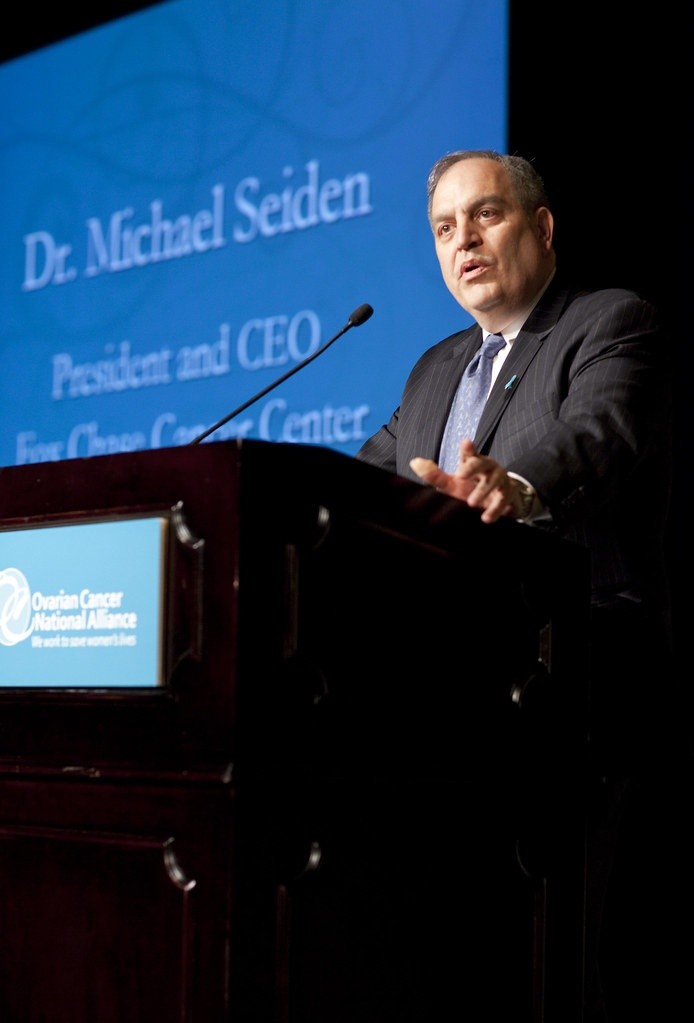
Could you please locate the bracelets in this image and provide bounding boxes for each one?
[517,483,534,517]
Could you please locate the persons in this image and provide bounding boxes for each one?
[349,149,654,535]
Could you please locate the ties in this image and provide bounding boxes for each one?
[439,334,507,475]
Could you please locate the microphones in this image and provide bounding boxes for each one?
[194,303,375,444]
